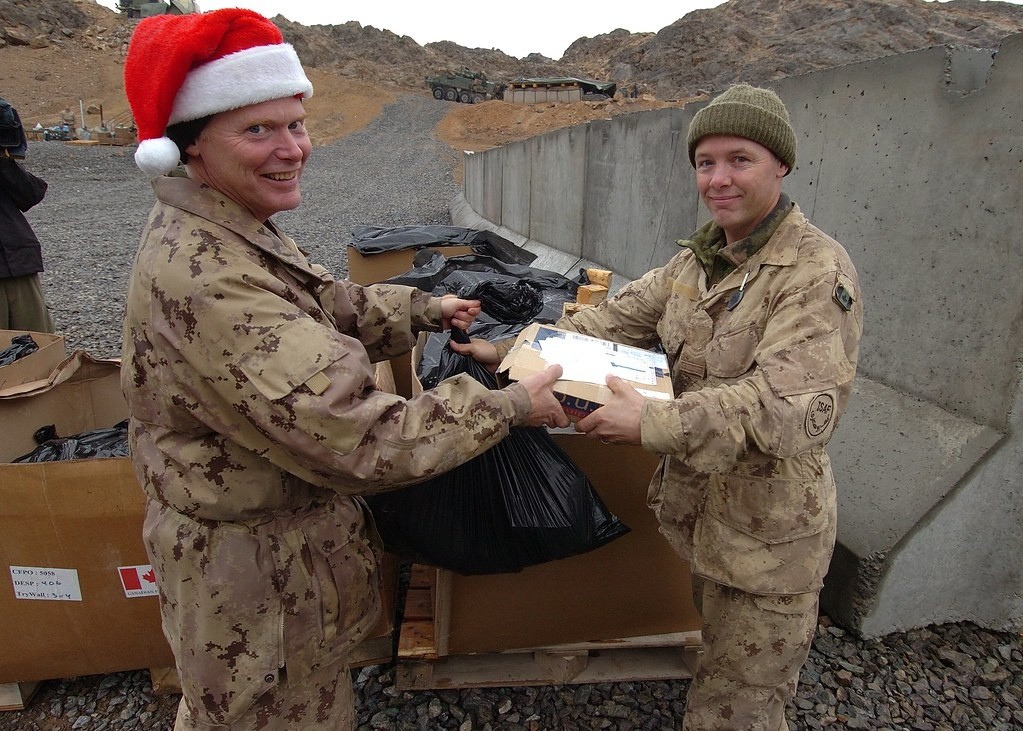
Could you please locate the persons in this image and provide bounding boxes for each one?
[449,84,864,731]
[0,152,56,334]
[119,8,568,731]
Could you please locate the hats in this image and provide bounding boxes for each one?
[686,82,797,178]
[122,7,313,174]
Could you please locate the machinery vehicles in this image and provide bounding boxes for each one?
[423,68,493,105]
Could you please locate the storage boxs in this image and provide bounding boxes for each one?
[0,329,66,391]
[496,323,674,422]
[347,244,475,285]
[0,348,176,684]
[411,330,703,657]
